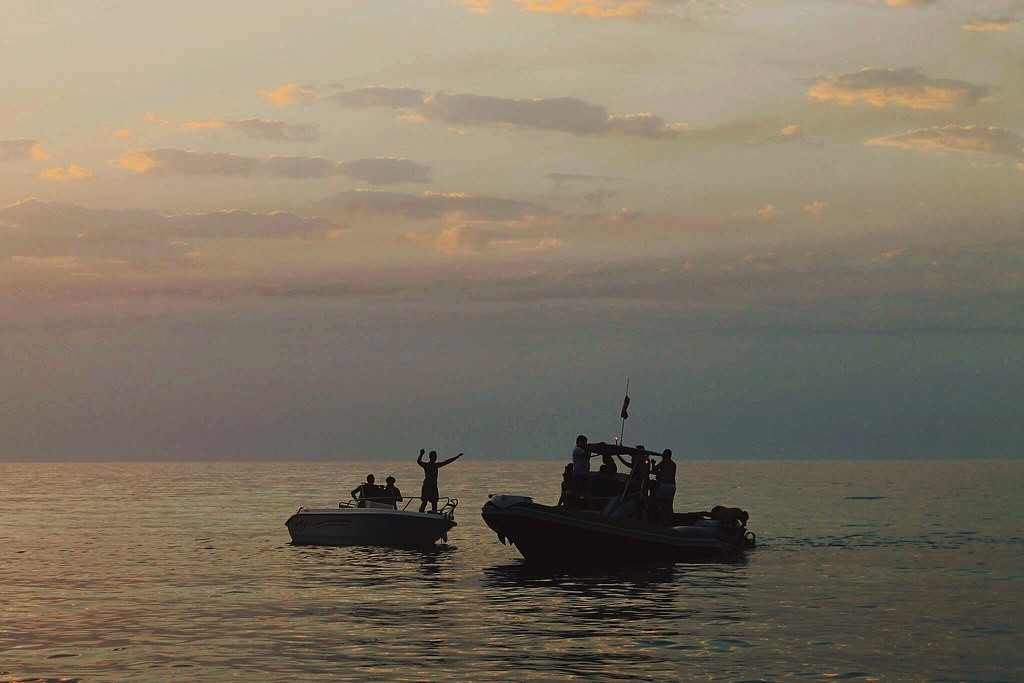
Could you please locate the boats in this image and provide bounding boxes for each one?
[285,496,458,545]
[481,378,756,564]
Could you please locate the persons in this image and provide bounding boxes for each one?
[350,474,404,509]
[557,434,677,517]
[416,448,463,513]
